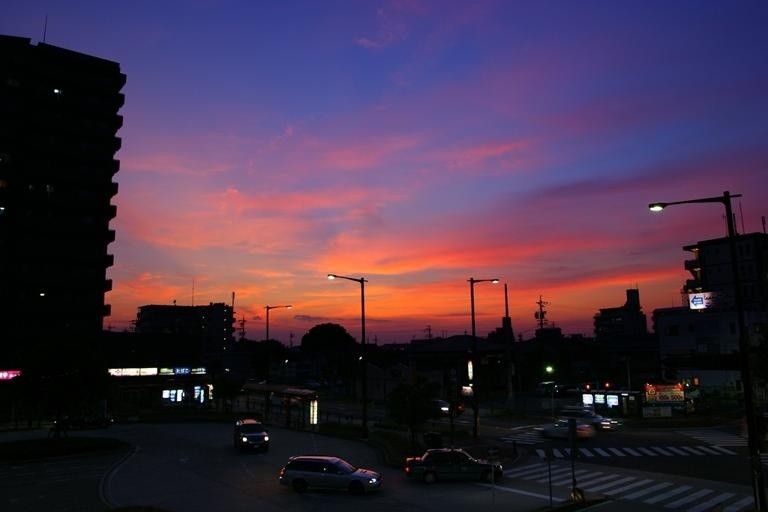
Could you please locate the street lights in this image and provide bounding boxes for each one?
[264,305,294,340]
[467,276,503,436]
[647,191,768,511]
[326,273,370,438]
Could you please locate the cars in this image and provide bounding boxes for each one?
[534,380,576,397]
[450,400,466,414]
[542,405,624,438]
[277,454,384,496]
[431,398,452,418]
[402,446,506,485]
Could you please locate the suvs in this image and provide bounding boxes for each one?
[231,417,273,455]
